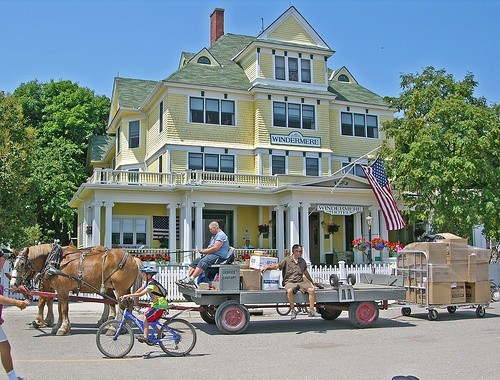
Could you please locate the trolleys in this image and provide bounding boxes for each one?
[394,251,492,320]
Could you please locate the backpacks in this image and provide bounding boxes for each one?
[147,279,168,297]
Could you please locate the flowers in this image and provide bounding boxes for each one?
[371,237,389,249]
[352,238,370,251]
[389,242,405,252]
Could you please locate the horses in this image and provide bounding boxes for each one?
[4,243,144,336]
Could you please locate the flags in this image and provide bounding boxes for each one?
[358,152,407,231]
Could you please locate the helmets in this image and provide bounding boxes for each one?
[140,264,158,273]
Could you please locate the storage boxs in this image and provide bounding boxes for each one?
[250,256,279,270]
[398,233,491,304]
[211,280,220,290]
[239,268,262,290]
[261,270,281,290]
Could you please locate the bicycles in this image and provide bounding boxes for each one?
[95,297,197,357]
[489,279,500,301]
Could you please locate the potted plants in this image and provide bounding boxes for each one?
[327,224,340,235]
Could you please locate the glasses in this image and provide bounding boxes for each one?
[296,250,303,253]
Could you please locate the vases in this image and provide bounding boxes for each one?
[359,246,366,251]
[377,247,383,250]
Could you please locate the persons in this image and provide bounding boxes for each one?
[178,220,230,283]
[242,240,253,248]
[261,244,322,320]
[0,251,27,380]
[123,264,169,342]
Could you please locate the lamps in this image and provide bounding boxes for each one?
[366,214,374,230]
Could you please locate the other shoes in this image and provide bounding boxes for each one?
[182,278,194,284]
[157,333,166,339]
[291,310,297,319]
[178,276,187,282]
[135,336,148,342]
[308,310,322,317]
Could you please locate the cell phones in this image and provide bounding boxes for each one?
[24,299,29,303]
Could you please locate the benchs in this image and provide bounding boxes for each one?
[205,247,241,291]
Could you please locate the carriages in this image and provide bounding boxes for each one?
[6,240,408,336]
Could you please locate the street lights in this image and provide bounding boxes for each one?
[366,214,373,265]
